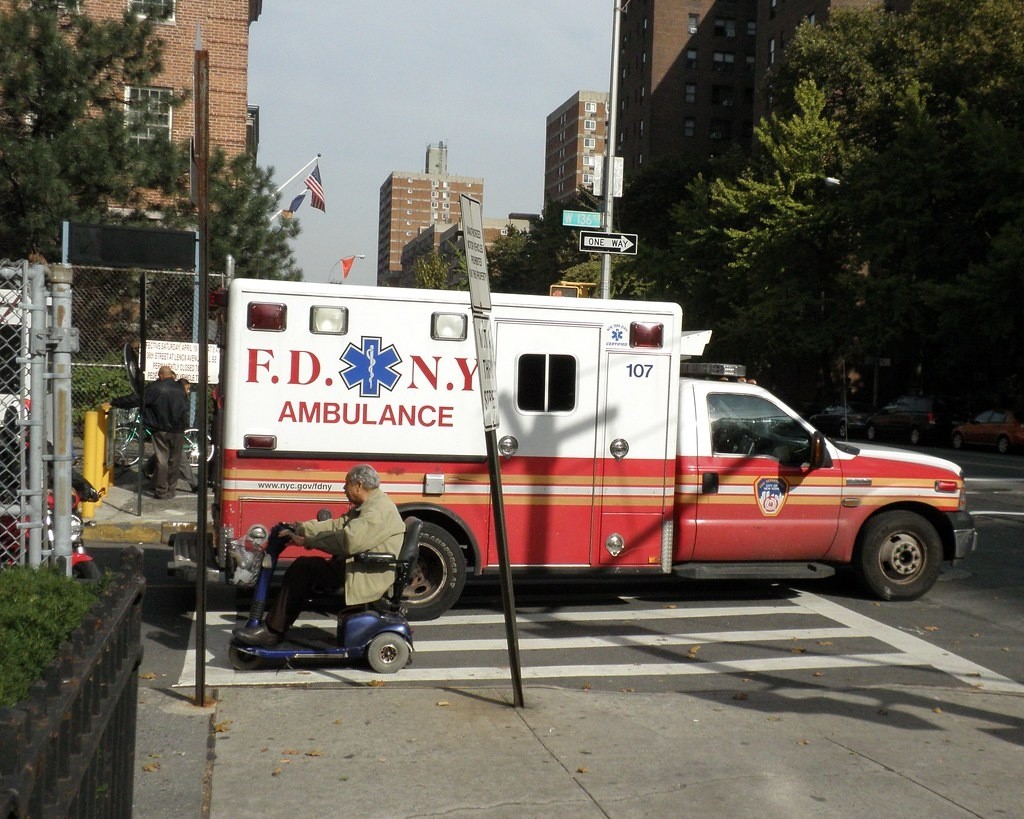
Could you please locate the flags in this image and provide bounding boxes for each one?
[289,164,325,212]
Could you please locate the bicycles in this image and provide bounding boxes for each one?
[114,408,215,468]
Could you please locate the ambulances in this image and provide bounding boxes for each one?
[210,276,984,623]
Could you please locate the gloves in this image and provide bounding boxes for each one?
[82,487,99,503]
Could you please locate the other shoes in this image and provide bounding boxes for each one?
[232,621,279,648]
[192,485,198,493]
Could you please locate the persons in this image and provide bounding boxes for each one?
[0,424,99,505]
[231,464,406,649]
[101,366,199,500]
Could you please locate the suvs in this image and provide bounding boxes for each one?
[862,394,954,445]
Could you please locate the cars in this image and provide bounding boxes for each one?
[948,409,1024,455]
[807,401,873,438]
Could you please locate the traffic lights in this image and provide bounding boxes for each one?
[548,285,588,297]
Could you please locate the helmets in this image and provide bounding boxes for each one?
[4,399,31,448]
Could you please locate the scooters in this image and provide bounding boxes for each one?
[228,515,425,672]
[0,484,101,584]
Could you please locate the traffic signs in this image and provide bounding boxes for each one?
[579,231,639,255]
